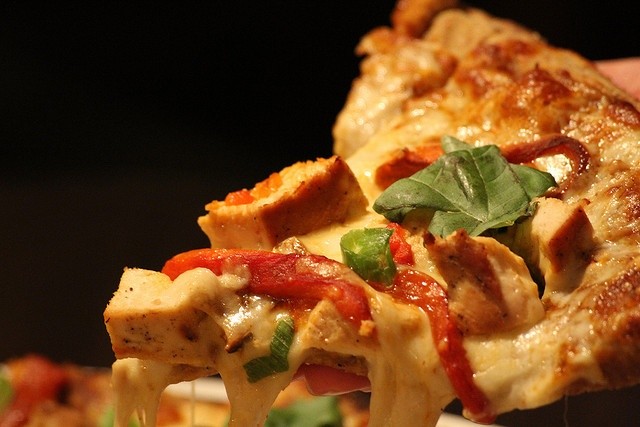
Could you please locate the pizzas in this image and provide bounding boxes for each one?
[104,1,640,427]
[0,346,404,427]
